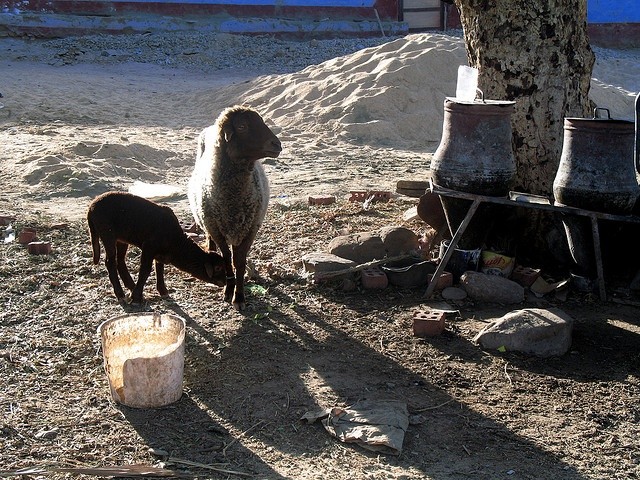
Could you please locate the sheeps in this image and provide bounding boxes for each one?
[87,190,228,307]
[185,105,282,311]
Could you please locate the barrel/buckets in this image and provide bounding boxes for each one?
[97,312,186,408]
